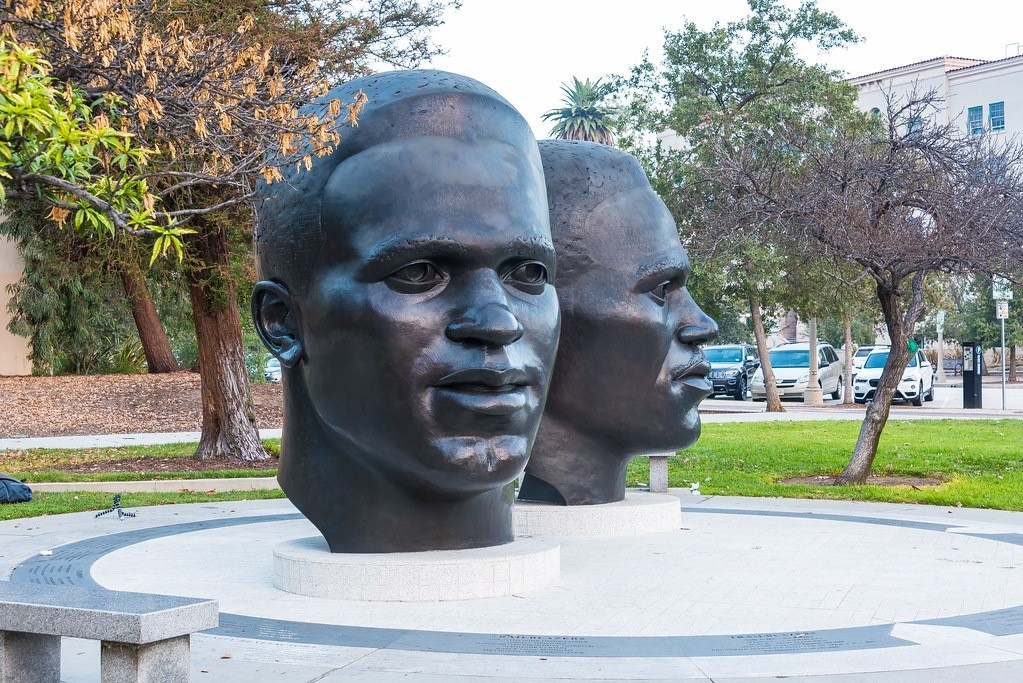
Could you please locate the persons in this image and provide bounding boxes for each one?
[515,140,718,506]
[251,70,562,554]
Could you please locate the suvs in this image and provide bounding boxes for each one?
[851,347,935,406]
[851,343,892,386]
[750,340,842,402]
[702,343,760,401]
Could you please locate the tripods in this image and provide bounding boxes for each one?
[94,495,136,521]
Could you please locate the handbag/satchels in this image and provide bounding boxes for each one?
[0,474,33,503]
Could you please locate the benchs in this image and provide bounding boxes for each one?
[0,580,219,683]
[931,358,964,377]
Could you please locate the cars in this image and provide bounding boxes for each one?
[253,357,281,385]
